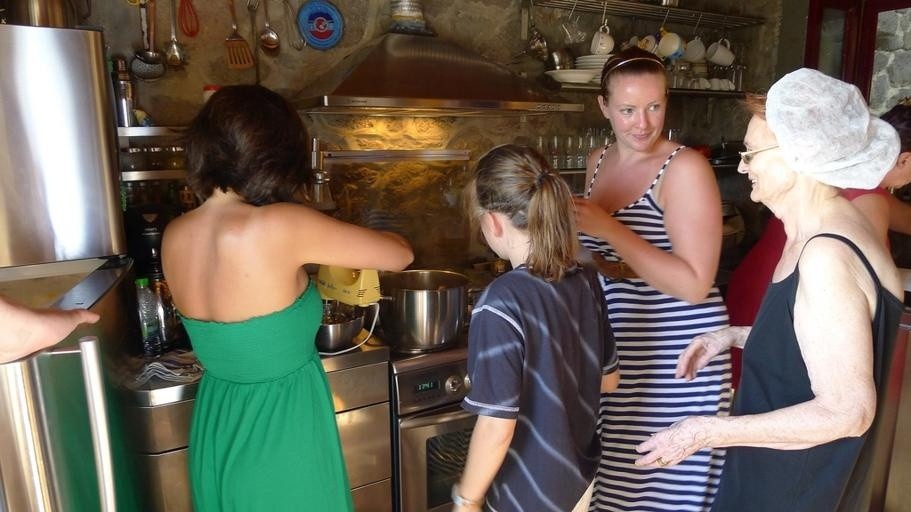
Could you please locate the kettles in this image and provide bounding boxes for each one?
[5,0,91,29]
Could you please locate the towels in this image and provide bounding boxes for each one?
[124,351,202,390]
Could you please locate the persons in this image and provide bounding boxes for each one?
[572,46,731,512]
[726,106,911,391]
[0,291,99,364]
[161,84,414,512]
[451,143,619,512]
[635,68,905,511]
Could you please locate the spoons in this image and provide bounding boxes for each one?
[259,0,280,49]
[135,0,163,65]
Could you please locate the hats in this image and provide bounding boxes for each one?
[765,68,901,190]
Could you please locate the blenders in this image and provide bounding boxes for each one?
[178,0,201,37]
[317,264,381,356]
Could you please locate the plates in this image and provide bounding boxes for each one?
[574,54,615,84]
[543,68,599,84]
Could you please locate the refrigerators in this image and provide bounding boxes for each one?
[0,23,127,266]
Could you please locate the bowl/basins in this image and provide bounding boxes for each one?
[547,47,574,69]
[314,304,364,353]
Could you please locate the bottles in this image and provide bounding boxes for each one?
[578,135,584,171]
[142,148,166,169]
[567,137,574,169]
[134,279,161,360]
[123,180,196,208]
[601,130,606,145]
[538,135,547,163]
[142,269,173,353]
[120,146,141,170]
[550,134,561,172]
[587,130,596,151]
[167,146,188,169]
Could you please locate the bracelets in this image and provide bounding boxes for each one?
[450,484,485,509]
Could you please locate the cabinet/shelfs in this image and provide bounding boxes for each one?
[106,328,390,512]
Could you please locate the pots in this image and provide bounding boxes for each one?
[379,269,473,355]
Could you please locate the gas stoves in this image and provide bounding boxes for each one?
[389,344,470,417]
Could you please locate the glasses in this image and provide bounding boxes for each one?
[739,145,781,164]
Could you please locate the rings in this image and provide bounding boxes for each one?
[656,457,668,468]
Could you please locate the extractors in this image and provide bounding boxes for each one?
[288,1,586,116]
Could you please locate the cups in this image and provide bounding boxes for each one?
[676,71,688,89]
[690,78,699,89]
[684,36,705,62]
[720,78,729,91]
[698,77,706,90]
[668,64,751,92]
[708,78,720,90]
[705,38,735,66]
[589,24,614,54]
[657,32,687,62]
[531,128,680,169]
